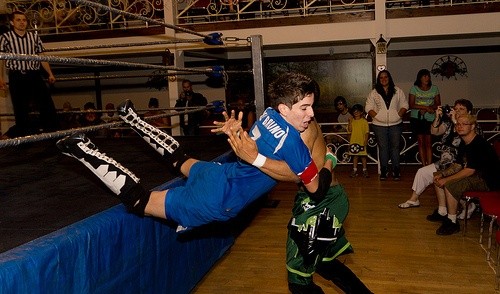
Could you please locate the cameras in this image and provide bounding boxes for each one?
[441,104,450,113]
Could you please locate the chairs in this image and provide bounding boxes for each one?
[463,191,500,277]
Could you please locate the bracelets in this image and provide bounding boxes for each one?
[431,122,442,128]
[440,172,445,177]
[251,153,267,169]
[324,146,338,170]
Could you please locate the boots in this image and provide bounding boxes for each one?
[116,99,182,159]
[55,131,147,206]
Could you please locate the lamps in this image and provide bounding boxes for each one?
[376,33,387,54]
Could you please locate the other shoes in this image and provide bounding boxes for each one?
[380,170,389,180]
[361,170,370,178]
[392,170,400,180]
[436,218,460,236]
[426,209,448,223]
[350,170,359,177]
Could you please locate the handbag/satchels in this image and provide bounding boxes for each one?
[417,111,428,132]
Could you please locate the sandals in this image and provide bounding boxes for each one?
[458,202,476,220]
[398,200,420,209]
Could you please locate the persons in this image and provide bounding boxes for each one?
[100,103,119,124]
[175,80,208,137]
[364,70,408,181]
[0,10,64,139]
[334,96,354,134]
[143,97,172,137]
[397,98,500,235]
[211,73,372,294]
[77,101,106,127]
[347,104,371,178]
[409,69,441,166]
[58,102,77,125]
[56,71,338,236]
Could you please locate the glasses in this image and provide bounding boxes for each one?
[337,102,342,105]
[454,123,472,127]
